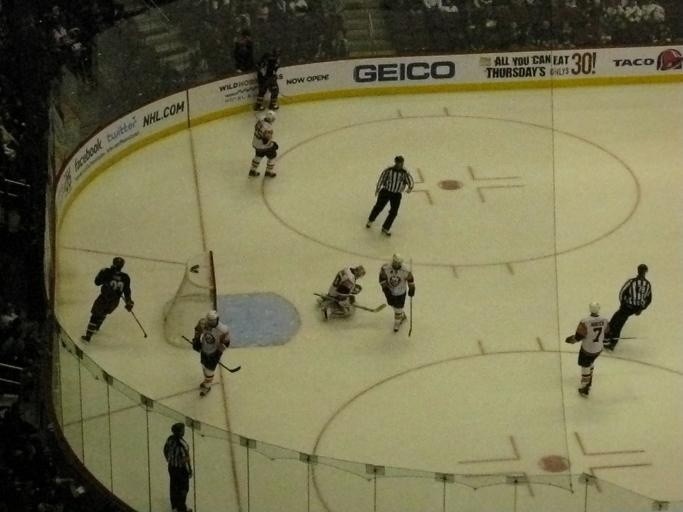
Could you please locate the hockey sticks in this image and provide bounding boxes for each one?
[181,335,241,372]
[565,333,645,343]
[311,291,386,313]
[119,294,148,339]
[406,258,412,336]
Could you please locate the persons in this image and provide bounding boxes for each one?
[163,424,196,512]
[567,300,609,395]
[81,256,136,342]
[365,156,415,236]
[247,109,281,178]
[379,252,417,332]
[603,264,651,348]
[102,1,682,120]
[191,308,232,397]
[0,1,102,512]
[320,264,367,322]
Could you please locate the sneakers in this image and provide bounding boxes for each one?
[366,220,372,228]
[249,170,260,177]
[382,226,391,235]
[199,378,211,396]
[82,336,90,341]
[255,102,280,111]
[579,388,588,395]
[265,171,276,178]
[394,320,401,331]
[325,306,332,321]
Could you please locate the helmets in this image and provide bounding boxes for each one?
[590,300,600,314]
[111,257,124,272]
[637,264,648,275]
[171,423,184,437]
[206,309,219,326]
[355,265,365,278]
[392,253,404,268]
[265,110,276,121]
